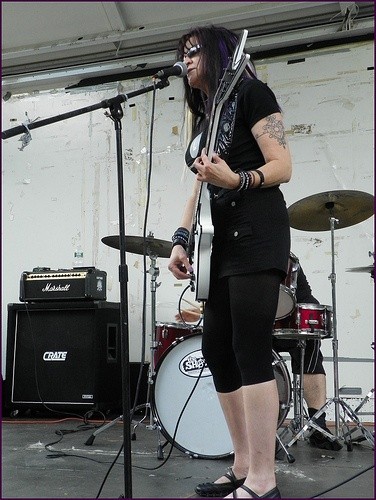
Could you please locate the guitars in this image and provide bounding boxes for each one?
[187,27,250,302]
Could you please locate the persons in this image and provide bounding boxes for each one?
[168,24,293,499]
[174,251,343,452]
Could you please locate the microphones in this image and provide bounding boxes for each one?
[153,61,187,79]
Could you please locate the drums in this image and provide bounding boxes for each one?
[275,251,300,320]
[153,321,203,370]
[148,331,292,460]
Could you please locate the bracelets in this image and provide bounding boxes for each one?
[232,168,255,193]
[251,169,264,190]
[172,227,189,251]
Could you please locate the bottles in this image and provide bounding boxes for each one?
[73,242,84,267]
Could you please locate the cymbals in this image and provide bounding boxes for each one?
[101,235,174,258]
[271,302,333,342]
[286,190,376,232]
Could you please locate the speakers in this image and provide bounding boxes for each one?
[4,300,121,416]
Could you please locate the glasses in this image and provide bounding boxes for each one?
[187,43,202,58]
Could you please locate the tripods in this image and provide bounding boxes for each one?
[274,204,374,460]
[85,243,164,460]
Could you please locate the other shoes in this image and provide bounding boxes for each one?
[194,467,247,497]
[310,428,342,451]
[225,484,281,500]
